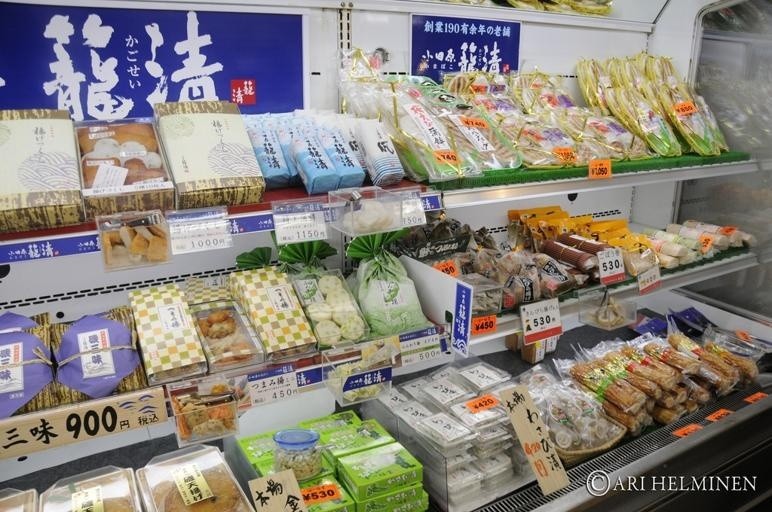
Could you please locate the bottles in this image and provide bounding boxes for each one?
[271,429,323,484]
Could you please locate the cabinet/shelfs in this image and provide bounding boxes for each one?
[2,0,772,511]
